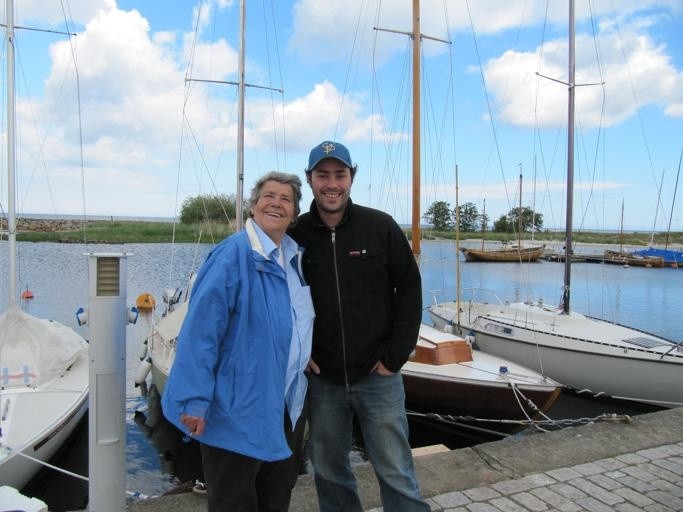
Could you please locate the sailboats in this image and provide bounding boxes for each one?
[137,0,291,448]
[428,3,682,408]
[330,1,565,442]
[0,0,88,508]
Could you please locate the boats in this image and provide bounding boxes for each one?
[457,151,682,269]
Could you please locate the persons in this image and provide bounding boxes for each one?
[286,141,430,511]
[159,172,316,511]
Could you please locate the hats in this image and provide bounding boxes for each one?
[307,141,351,173]
